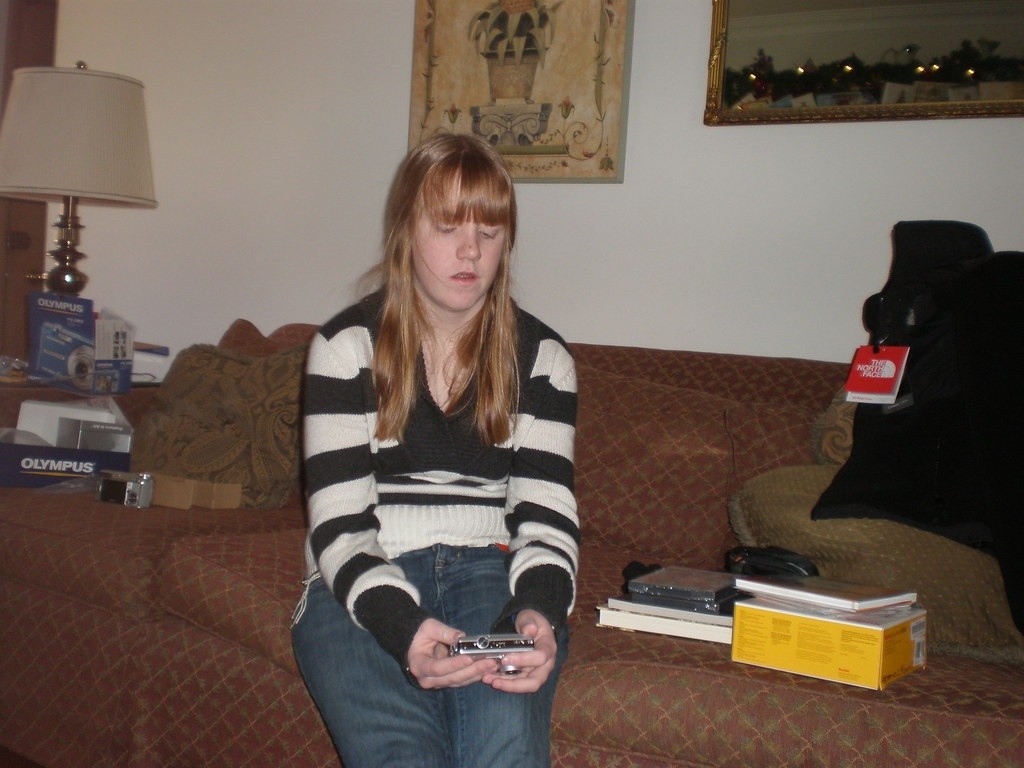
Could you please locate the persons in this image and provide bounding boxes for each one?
[291,134,579,768]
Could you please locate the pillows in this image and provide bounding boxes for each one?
[130,343,311,510]
[724,401,828,482]
[576,361,740,573]
[809,385,857,466]
[727,464,1024,666]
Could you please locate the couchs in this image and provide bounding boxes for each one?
[0,320,1024,768]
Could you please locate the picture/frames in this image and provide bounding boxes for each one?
[407,0,636,185]
[702,1,1024,126]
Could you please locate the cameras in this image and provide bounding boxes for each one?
[97,473,154,508]
[449,632,534,676]
[36,322,95,392]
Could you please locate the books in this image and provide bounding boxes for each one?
[736,575,916,614]
[596,604,732,645]
[608,594,756,627]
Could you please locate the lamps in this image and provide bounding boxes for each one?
[0,60,158,301]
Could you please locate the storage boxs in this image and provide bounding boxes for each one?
[0,292,169,489]
[730,598,927,691]
